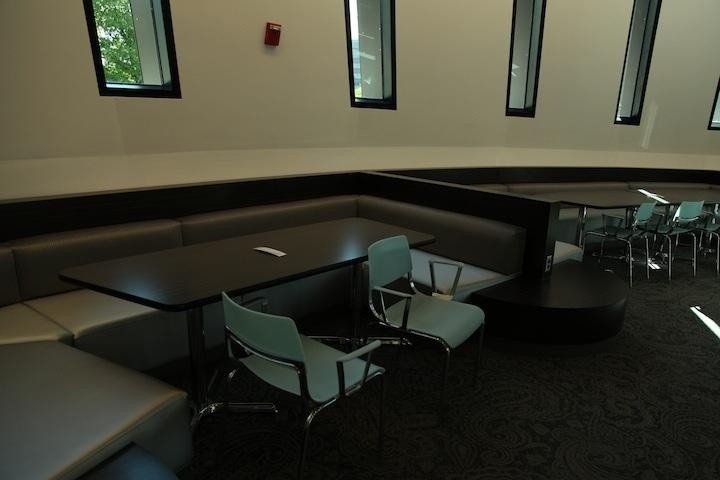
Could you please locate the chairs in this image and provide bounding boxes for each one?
[211,288,397,478]
[582,199,719,289]
[358,229,488,419]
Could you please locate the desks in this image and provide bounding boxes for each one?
[538,188,720,262]
[56,215,440,444]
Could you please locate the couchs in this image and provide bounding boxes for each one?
[419,167,717,278]
[0,189,527,478]
